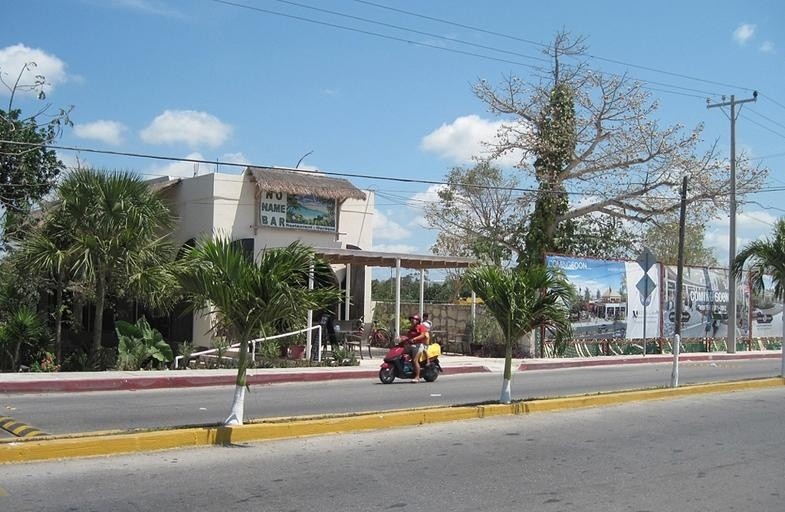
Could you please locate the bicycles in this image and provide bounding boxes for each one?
[356,315,392,348]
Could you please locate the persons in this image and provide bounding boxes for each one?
[404,313,433,383]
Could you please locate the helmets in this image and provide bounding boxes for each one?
[409,313,421,322]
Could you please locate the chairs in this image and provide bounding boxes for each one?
[345,322,375,360]
[445,333,467,357]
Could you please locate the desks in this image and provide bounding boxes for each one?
[335,329,362,358]
[430,330,448,354]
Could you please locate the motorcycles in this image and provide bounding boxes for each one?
[379,340,443,384]
